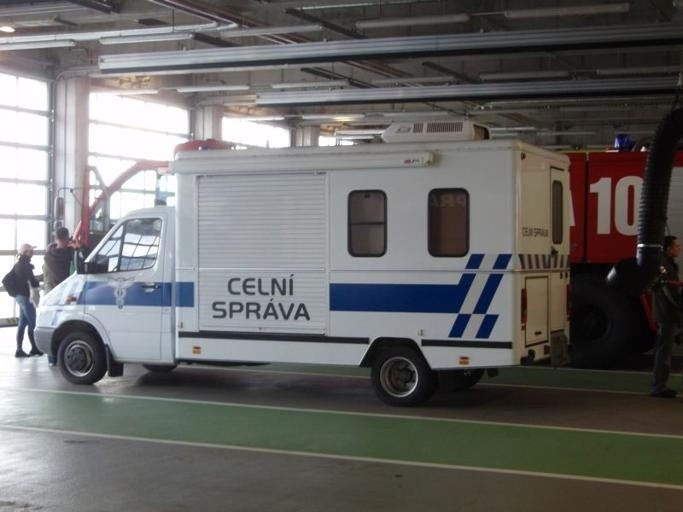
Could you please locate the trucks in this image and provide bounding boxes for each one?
[32,134,571,405]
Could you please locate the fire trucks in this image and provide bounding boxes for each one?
[73,135,682,330]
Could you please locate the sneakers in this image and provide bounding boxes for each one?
[16,350,43,357]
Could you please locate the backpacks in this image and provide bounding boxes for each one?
[2,266,18,297]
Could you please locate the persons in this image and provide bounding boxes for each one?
[651,235,683,397]
[42,228,90,366]
[14,244,44,357]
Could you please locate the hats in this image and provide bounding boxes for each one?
[18,244,37,255]
